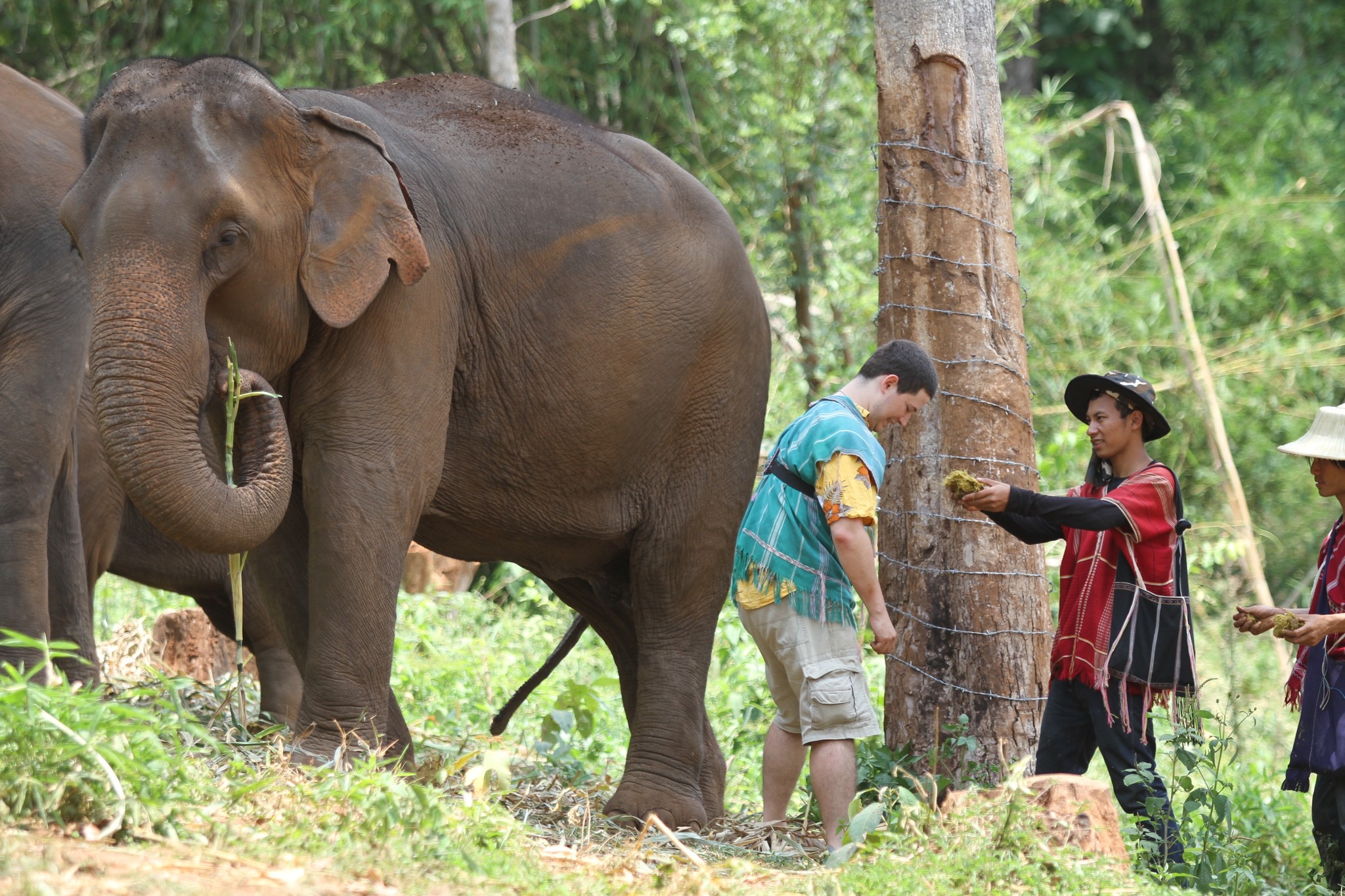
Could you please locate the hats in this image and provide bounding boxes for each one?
[1064,370,1172,445]
[1276,403,1345,461]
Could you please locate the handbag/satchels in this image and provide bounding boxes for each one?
[1094,582,1204,747]
[1281,657,1345,793]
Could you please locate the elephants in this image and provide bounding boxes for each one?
[0,55,772,845]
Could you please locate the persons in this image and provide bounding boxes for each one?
[732,339,939,867]
[950,369,1200,867]
[1232,407,1345,896]
